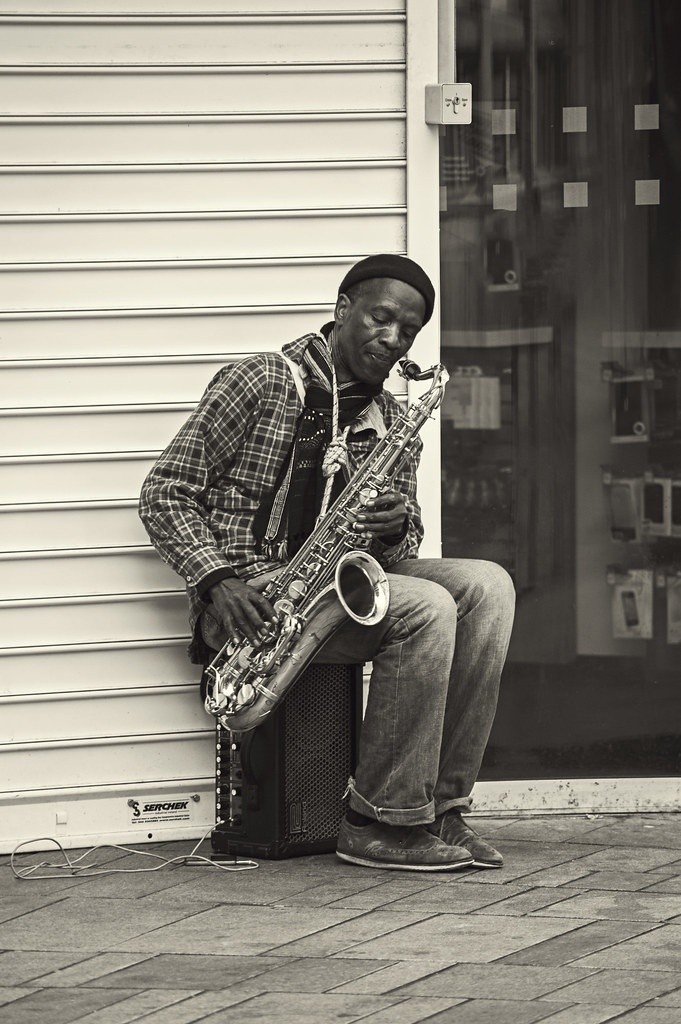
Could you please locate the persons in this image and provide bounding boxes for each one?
[137,253,518,872]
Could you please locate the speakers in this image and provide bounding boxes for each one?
[212,662,363,860]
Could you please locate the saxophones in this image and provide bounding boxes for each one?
[200,355,451,734]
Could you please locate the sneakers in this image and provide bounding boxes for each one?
[336,814,475,871]
[423,809,504,868]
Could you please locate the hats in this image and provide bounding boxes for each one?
[338,254,435,328]
